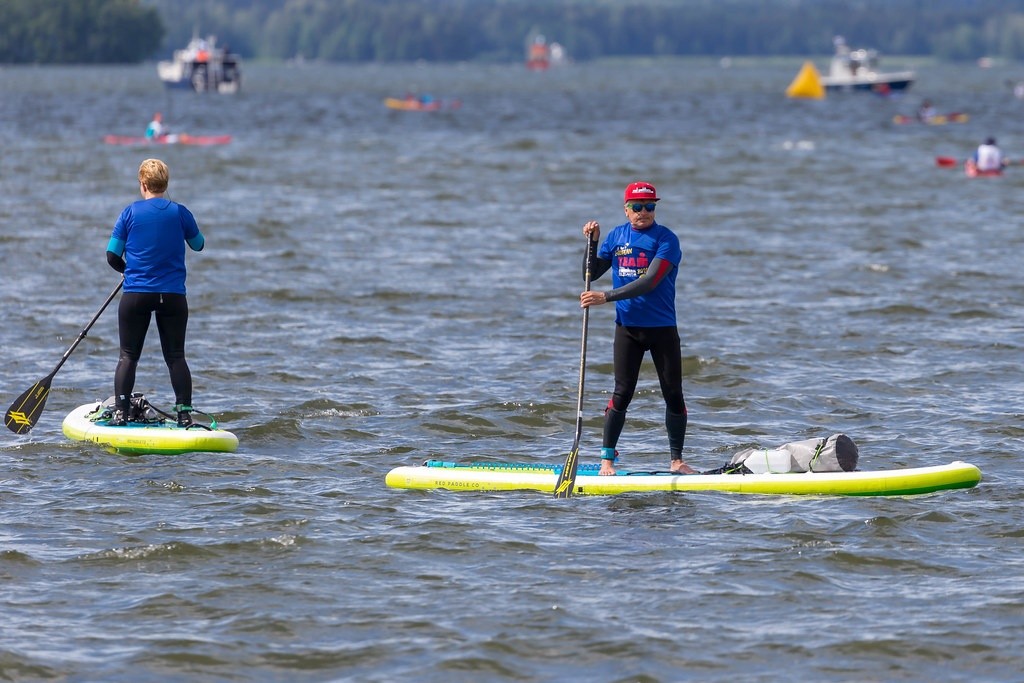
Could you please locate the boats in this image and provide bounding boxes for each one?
[103,132,233,147]
[156,34,247,98]
[965,156,1006,180]
[382,96,464,114]
[814,34,918,96]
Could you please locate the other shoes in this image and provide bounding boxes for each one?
[177,412,192,427]
[108,411,126,426]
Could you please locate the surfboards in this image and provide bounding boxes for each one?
[60,399,241,456]
[382,459,984,499]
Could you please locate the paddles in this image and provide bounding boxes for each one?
[552,226,597,500]
[3,278,125,436]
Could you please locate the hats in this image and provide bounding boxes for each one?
[624,182,660,202]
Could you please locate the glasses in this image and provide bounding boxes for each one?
[625,203,657,213]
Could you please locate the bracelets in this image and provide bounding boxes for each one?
[603,294,607,302]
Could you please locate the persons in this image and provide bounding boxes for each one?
[144,113,166,137]
[974,137,1001,171]
[106,159,205,428]
[916,100,936,119]
[580,182,698,476]
